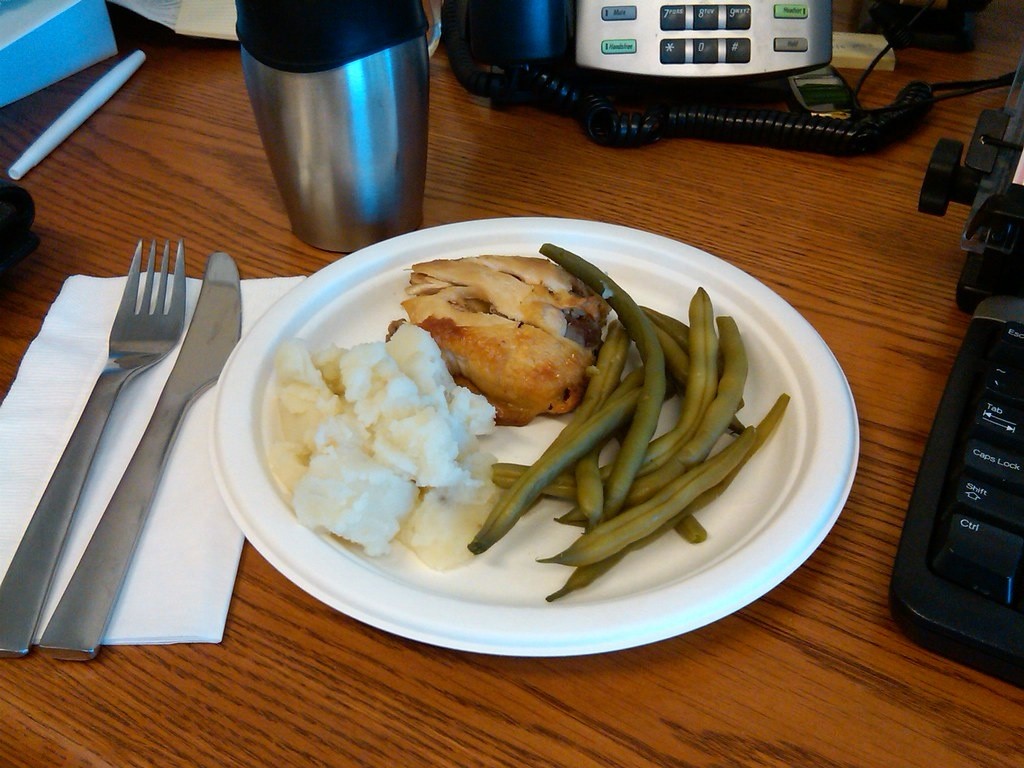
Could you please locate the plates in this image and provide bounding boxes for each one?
[209,216,857,657]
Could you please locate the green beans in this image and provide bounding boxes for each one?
[466,241,790,604]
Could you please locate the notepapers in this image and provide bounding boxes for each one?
[831,32,896,73]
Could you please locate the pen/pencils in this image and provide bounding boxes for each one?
[7,49,148,182]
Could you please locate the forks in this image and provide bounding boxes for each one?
[0,235,188,661]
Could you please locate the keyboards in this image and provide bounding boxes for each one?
[889,293,1024,688]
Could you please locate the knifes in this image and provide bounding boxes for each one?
[38,254,243,661]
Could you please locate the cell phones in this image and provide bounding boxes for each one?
[785,63,863,113]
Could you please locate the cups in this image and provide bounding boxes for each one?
[234,1,430,254]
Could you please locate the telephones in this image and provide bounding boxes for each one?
[440,0,833,97]
